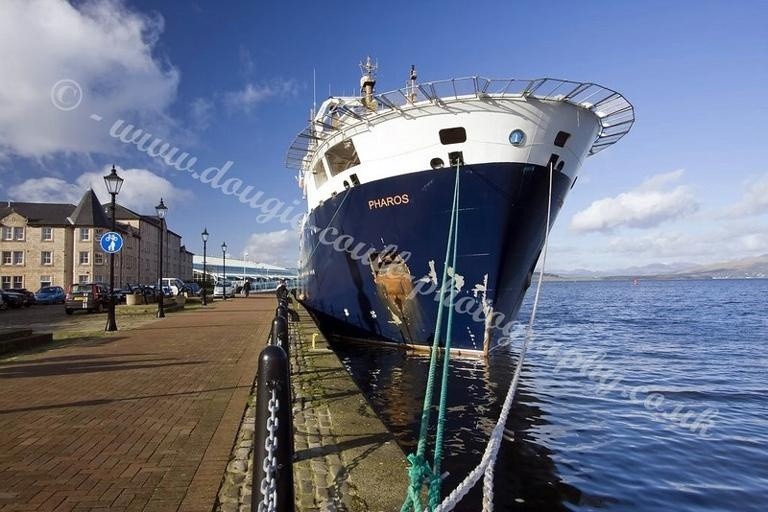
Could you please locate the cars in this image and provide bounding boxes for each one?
[0,283,173,315]
[186,283,203,297]
[214,281,235,298]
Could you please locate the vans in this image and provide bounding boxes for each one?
[158,277,186,296]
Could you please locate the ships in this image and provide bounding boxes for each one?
[285,54,635,367]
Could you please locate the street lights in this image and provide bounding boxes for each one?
[155,197,168,318]
[243,253,248,283]
[104,164,124,331]
[202,227,209,305]
[221,242,227,300]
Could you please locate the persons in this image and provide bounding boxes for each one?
[242,279,252,298]
